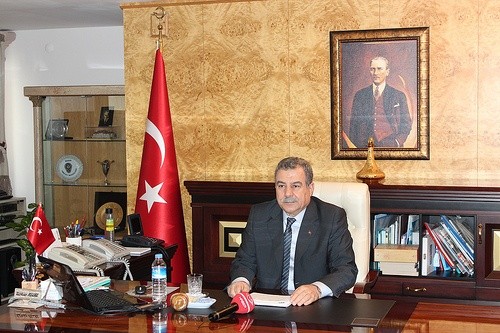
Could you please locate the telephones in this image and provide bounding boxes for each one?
[47,244,106,270]
[86,239,130,261]
[121,236,165,246]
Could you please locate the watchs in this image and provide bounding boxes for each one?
[313,284,321,294]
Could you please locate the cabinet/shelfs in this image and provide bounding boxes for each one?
[0,199,24,302]
[184,177,500,302]
[23,85,127,232]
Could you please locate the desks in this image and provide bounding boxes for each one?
[0,244,419,333]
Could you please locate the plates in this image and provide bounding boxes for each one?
[187,297,216,309]
[55,154,83,183]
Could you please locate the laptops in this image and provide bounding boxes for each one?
[37,255,163,313]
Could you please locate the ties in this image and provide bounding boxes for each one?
[375,87,380,101]
[280,218,296,291]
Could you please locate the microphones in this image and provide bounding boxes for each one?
[208,292,255,322]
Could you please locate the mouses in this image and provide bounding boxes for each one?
[135,286,147,294]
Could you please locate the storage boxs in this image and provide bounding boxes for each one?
[374,244,419,261]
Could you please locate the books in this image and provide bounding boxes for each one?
[372,214,475,277]
[41,276,114,301]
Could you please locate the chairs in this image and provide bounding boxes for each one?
[311,181,380,299]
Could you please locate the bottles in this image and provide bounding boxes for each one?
[103,208,115,242]
[153,298,169,333]
[151,254,168,297]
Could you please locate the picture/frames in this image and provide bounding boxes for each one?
[45,119,69,137]
[330,27,430,161]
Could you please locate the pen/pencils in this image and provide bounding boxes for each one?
[63,217,86,239]
[22,267,37,282]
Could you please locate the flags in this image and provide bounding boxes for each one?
[135,50,191,322]
[27,204,55,255]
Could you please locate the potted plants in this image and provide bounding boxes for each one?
[6,203,43,290]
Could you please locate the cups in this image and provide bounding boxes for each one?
[187,273,203,295]
[66,236,83,247]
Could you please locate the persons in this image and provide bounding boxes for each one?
[227,157,359,308]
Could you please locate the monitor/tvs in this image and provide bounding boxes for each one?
[127,214,143,236]
